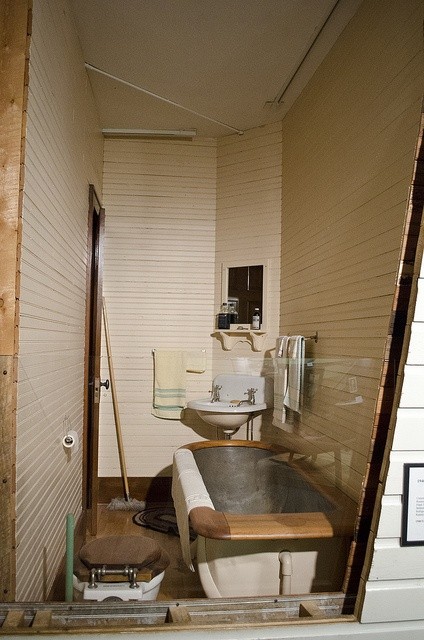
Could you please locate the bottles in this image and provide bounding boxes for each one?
[252,308,260,330]
[222,303,228,313]
[228,304,236,312]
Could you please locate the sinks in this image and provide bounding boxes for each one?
[187,398,273,434]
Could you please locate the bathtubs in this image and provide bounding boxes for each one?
[173,440,357,598]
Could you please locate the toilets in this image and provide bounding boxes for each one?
[69,535,170,601]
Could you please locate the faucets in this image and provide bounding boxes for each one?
[211,384,222,402]
[239,389,258,405]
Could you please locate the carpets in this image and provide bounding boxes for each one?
[132,507,198,541]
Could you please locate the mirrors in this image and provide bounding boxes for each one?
[215,262,269,333]
